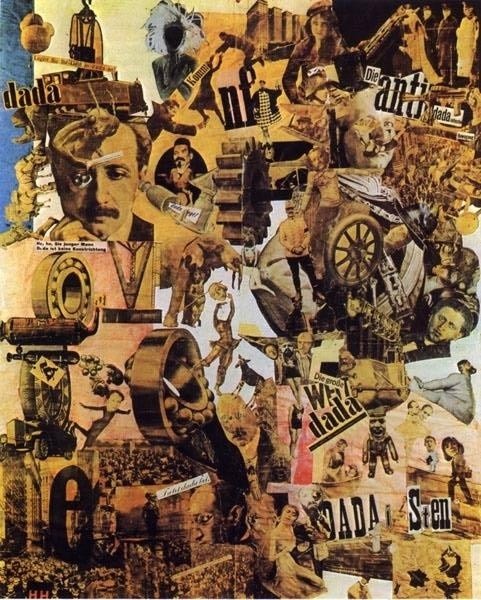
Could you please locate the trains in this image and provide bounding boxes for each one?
[32,64,149,121]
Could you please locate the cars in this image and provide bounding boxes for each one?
[1,416,78,462]
